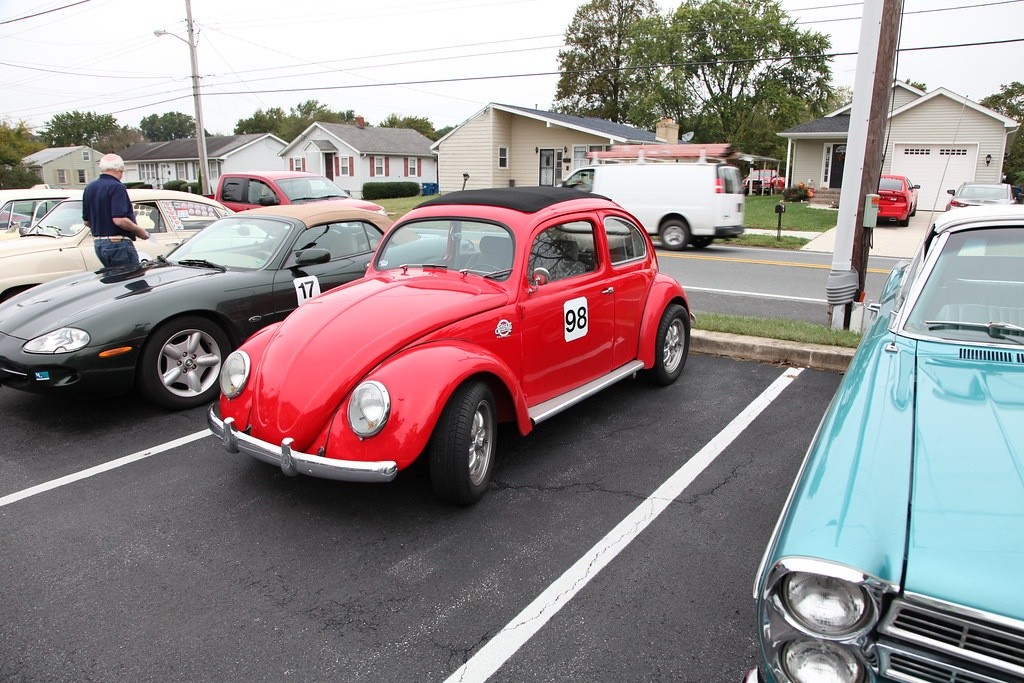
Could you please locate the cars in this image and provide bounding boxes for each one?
[0,187,74,229]
[206,187,697,506]
[0,204,480,408]
[876,175,920,226]
[750,200,1024,682]
[1,188,273,291]
[943,180,1018,212]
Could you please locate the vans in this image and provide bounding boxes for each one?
[550,162,748,253]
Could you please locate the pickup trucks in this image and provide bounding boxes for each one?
[742,163,787,194]
[177,170,387,216]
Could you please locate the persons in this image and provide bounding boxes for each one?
[82,153,150,267]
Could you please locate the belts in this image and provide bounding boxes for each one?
[93,236,132,243]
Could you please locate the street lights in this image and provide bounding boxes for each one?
[153,28,211,192]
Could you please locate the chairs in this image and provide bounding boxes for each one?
[529,240,585,283]
[465,236,514,278]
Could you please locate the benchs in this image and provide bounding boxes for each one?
[304,230,358,258]
